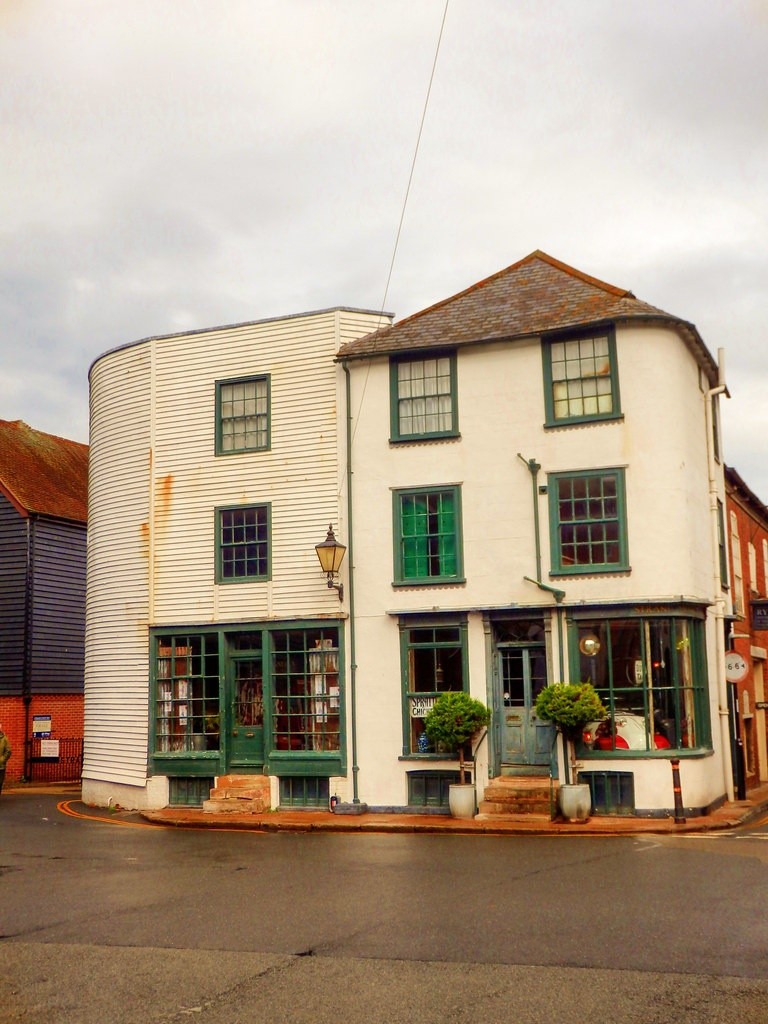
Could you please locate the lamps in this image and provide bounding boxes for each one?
[314,522,347,602]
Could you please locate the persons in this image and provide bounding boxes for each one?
[0,723,11,795]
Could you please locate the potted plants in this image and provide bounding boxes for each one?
[421,690,492,821]
[536,676,609,824]
